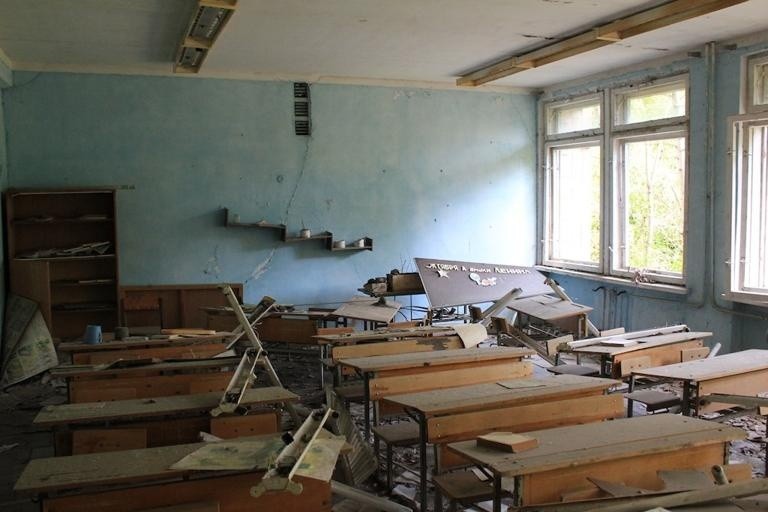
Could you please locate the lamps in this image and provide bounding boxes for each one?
[171,0,237,74]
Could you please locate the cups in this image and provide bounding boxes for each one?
[353,239,364,247]
[300,230,310,237]
[85,325,103,344]
[335,240,346,248]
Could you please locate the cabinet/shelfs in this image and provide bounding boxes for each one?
[223,207,373,253]
[6,187,124,343]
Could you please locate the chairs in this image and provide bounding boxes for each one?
[210,413,277,444]
[72,426,147,458]
[120,298,163,336]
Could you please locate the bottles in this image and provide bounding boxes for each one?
[233,212,240,223]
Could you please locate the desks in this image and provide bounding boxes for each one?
[14,426,350,510]
[34,386,299,440]
[59,332,236,365]
[199,306,335,344]
[50,355,265,404]
